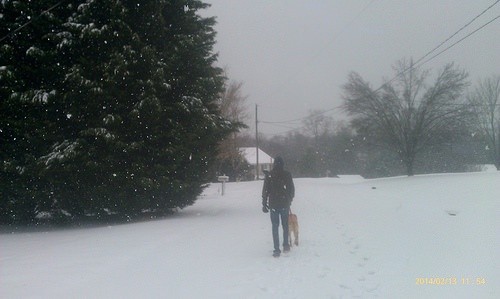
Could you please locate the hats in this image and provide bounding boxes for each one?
[273,156,284,165]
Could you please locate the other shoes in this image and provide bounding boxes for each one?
[273,243,291,258]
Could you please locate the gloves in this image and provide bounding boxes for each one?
[263,205,270,214]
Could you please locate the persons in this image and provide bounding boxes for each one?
[262,156,295,257]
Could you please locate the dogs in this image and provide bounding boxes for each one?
[287,214,299,247]
